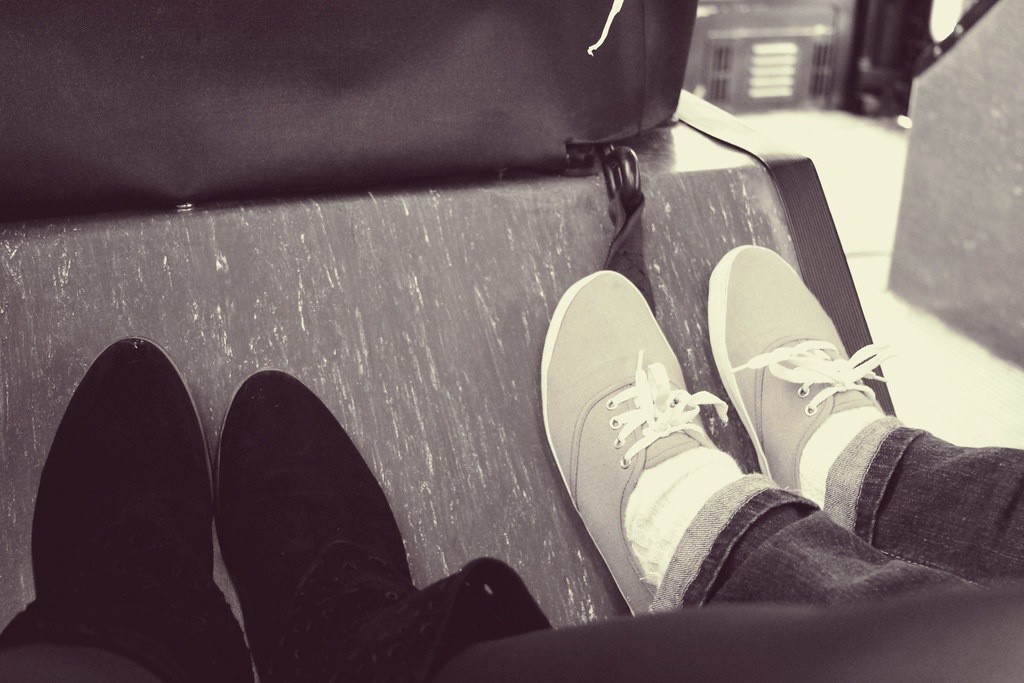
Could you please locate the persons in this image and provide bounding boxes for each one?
[0,337,1024,683]
[540,244,1024,615]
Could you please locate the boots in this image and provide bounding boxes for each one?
[0,337,213,682]
[214,371,553,683]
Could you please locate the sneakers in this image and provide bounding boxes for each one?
[540,270,744,619]
[707,242,897,500]
